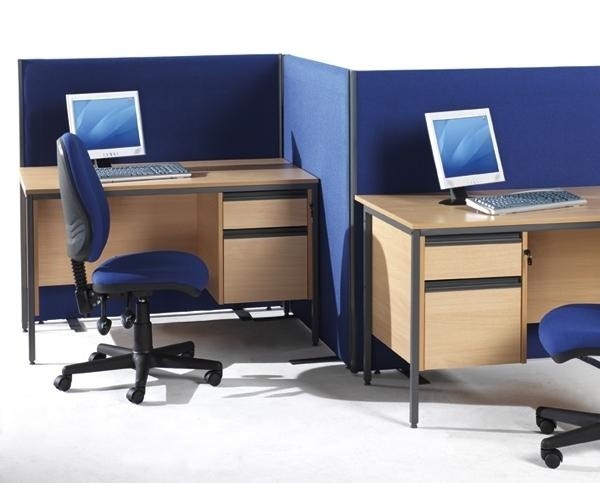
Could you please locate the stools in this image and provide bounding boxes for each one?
[536,303,600,468]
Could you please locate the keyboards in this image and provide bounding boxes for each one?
[464,187,589,217]
[95,162,192,184]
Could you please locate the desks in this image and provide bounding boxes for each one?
[354,186,600,428]
[20,156,321,366]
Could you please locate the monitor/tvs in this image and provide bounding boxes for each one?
[422,106,506,206]
[65,89,148,168]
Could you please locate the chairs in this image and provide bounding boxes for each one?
[53,134,222,404]
